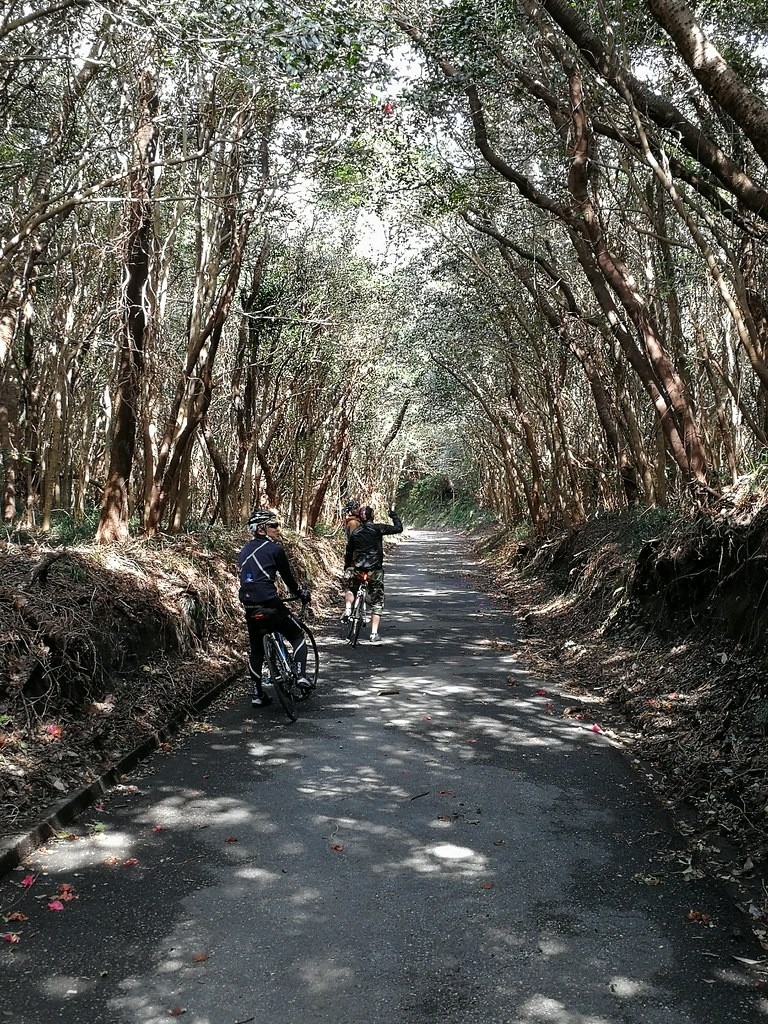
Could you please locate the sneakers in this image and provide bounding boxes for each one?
[340,609,351,624]
[370,633,381,642]
[296,676,316,689]
[252,692,273,707]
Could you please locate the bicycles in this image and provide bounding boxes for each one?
[262,592,320,723]
[342,563,381,649]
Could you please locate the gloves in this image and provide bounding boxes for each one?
[388,507,397,518]
[298,589,310,601]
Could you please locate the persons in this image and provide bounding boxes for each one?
[235,510,313,707]
[339,505,404,644]
[343,502,362,539]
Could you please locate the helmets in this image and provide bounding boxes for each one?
[359,506,374,522]
[345,501,360,512]
[247,510,277,543]
